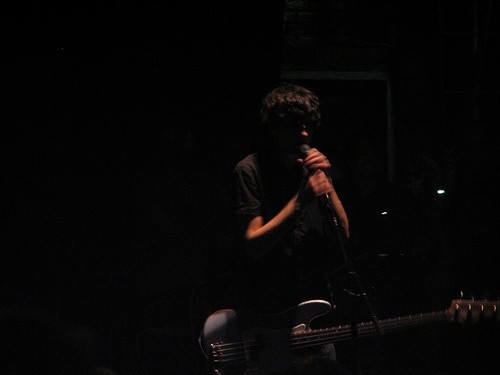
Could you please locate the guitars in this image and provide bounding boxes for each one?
[201,300,499,375]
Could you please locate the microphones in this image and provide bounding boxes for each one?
[300,144,332,206]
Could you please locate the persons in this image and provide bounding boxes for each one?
[234,85,351,314]
